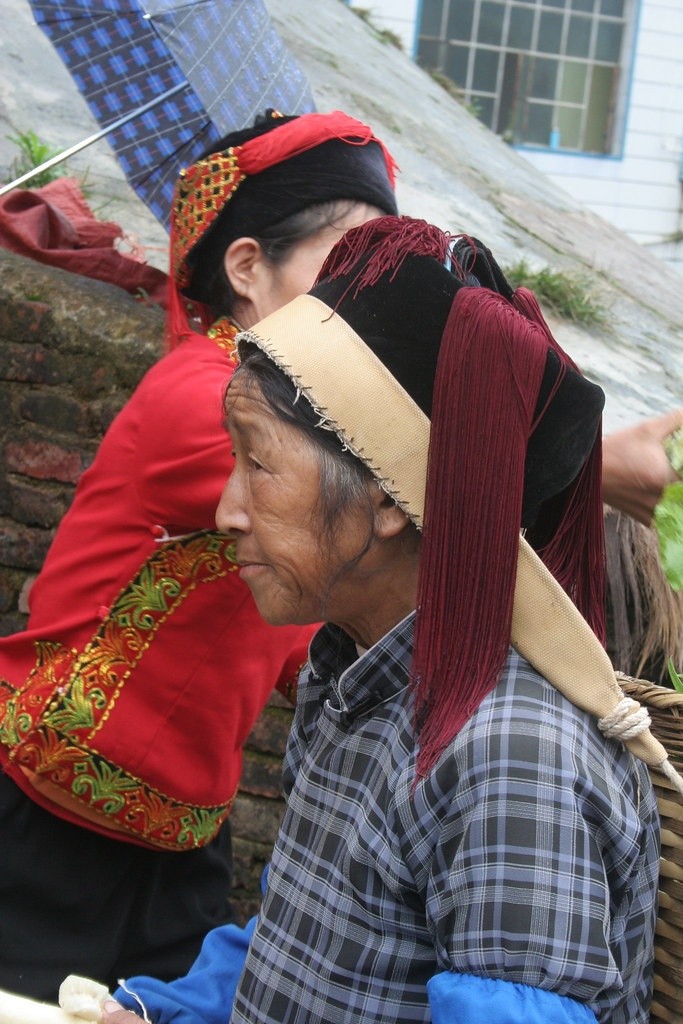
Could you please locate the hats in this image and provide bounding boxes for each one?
[163,108,400,350]
[241,211,609,792]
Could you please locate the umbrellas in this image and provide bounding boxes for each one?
[0,1,317,236]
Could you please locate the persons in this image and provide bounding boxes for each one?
[1,107,683,1005]
[98,215,683,1024]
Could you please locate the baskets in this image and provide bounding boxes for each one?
[610,667,683,1024]
